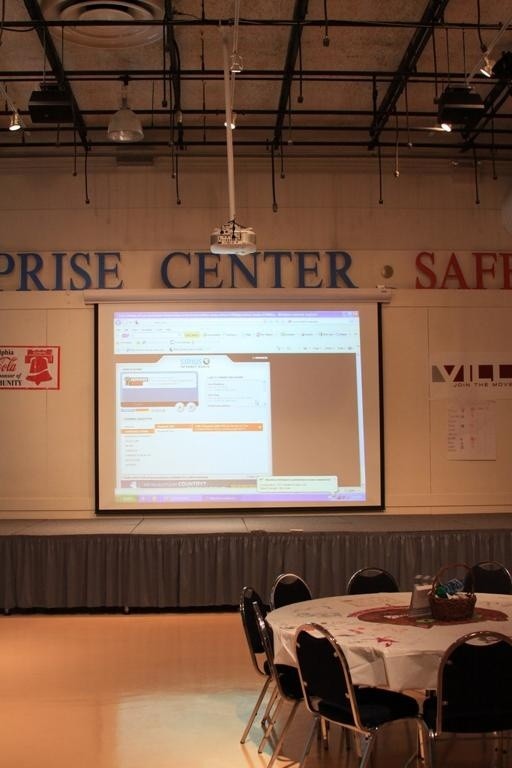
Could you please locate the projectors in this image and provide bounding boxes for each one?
[209,223,256,256]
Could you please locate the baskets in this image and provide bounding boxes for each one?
[427,563,477,621]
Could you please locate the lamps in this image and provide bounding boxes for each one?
[9,112,21,131]
[479,57,495,77]
[442,123,453,132]
[106,76,145,142]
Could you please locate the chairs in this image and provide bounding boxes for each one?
[239,586,330,744]
[293,621,429,768]
[251,601,351,768]
[347,567,400,594]
[270,573,313,611]
[421,631,512,768]
[461,560,512,595]
